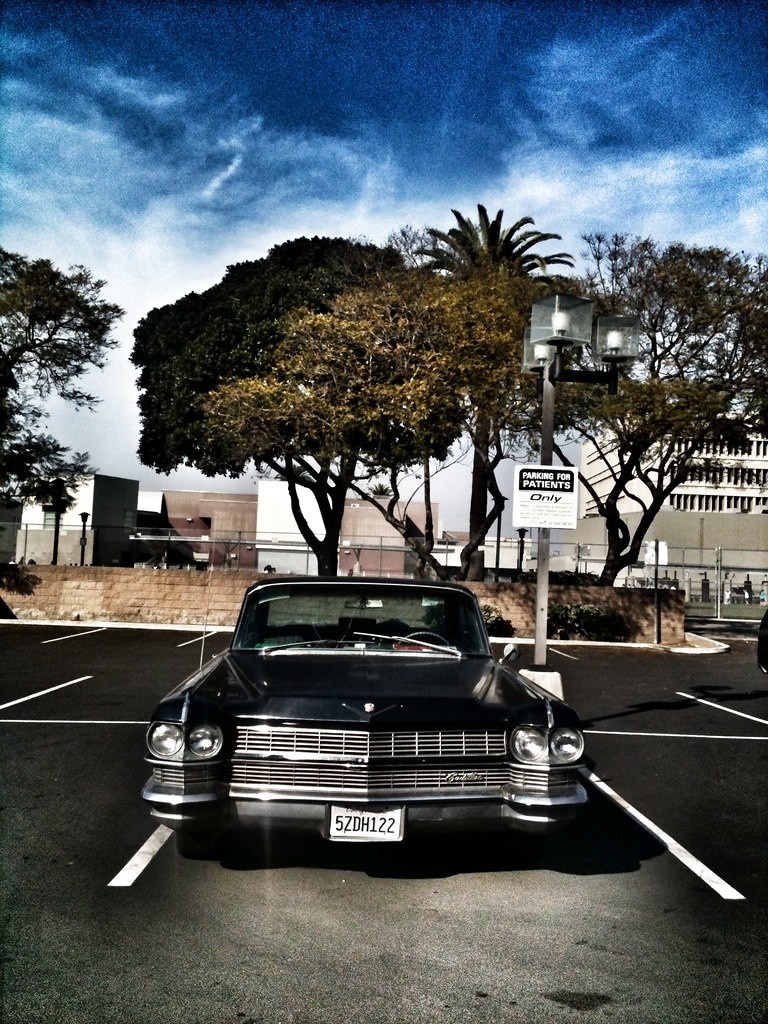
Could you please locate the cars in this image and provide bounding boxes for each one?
[139,575,587,855]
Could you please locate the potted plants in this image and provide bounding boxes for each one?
[535,603,631,644]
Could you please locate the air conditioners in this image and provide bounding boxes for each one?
[351,503,360,508]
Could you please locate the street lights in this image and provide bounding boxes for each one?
[515,527,529,575]
[78,511,91,566]
[519,292,640,700]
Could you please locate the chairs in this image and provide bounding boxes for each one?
[267,617,453,652]
